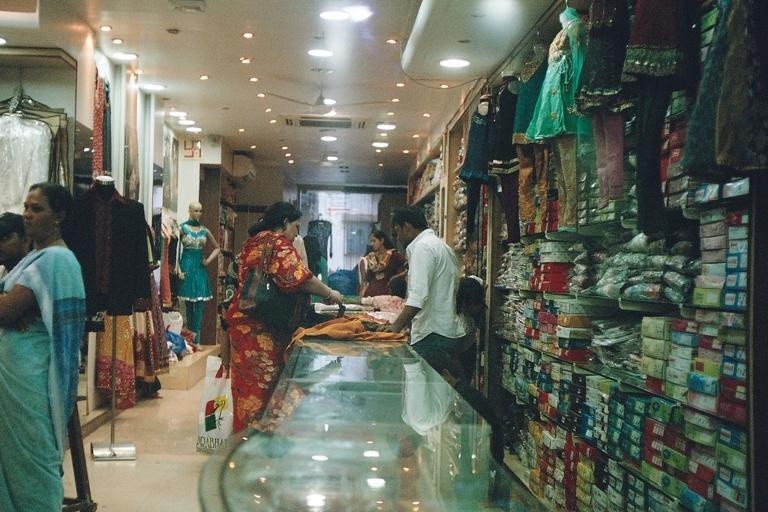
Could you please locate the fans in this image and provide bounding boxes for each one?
[261,66,393,117]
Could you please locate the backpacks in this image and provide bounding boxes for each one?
[456,271,487,319]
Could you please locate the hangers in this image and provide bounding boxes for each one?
[5,85,30,114]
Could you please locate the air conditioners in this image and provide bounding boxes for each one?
[231,154,257,185]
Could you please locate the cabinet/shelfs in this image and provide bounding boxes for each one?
[405,0,768,512]
[198,163,237,345]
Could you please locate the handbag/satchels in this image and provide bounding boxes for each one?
[237,233,307,332]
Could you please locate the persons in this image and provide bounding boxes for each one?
[358,229,410,296]
[376,205,477,393]
[0,212,27,272]
[217,201,342,435]
[1,182,86,510]
[175,202,220,348]
